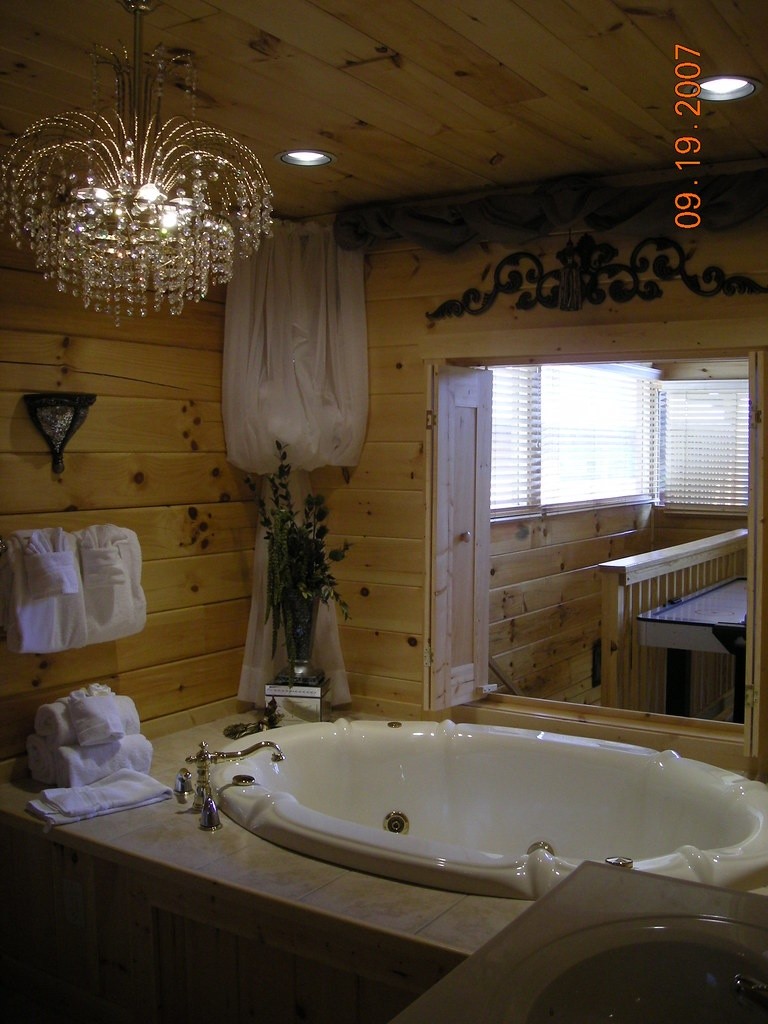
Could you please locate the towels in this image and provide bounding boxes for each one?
[3,522,152,654]
[25,679,155,785]
[26,767,175,828]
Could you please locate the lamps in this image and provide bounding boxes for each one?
[0,0,275,327]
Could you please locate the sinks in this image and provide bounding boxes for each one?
[479,911,768,1024]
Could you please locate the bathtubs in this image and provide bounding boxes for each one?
[204,714,768,903]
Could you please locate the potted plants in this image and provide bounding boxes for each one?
[244,440,351,684]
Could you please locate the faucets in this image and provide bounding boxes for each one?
[185,737,286,811]
[727,972,768,1013]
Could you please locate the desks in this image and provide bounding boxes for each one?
[637,577,746,721]
